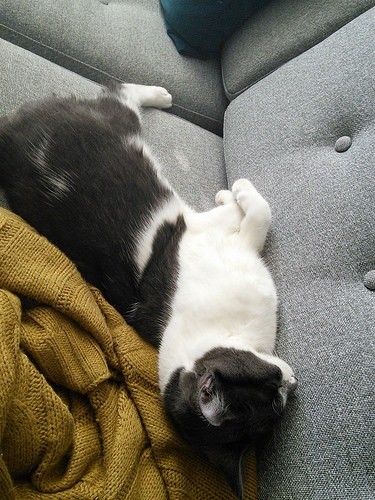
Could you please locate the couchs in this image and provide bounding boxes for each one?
[0,0,375,500]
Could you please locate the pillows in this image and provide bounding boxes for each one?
[160,0,270,60]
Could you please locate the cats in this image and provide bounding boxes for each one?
[0,80,299,499]
